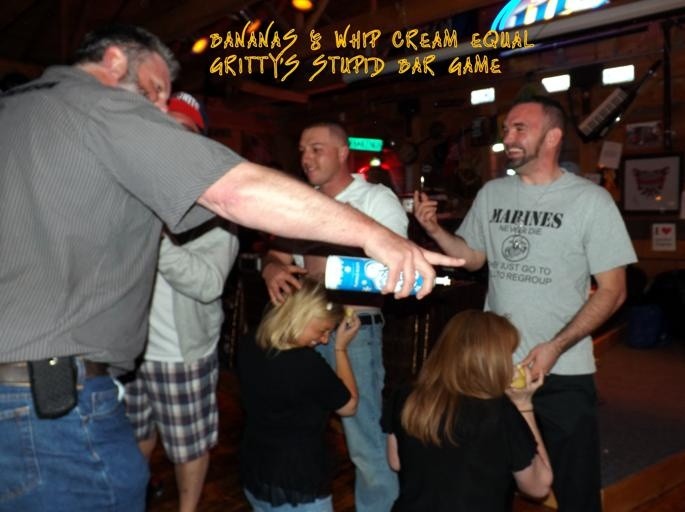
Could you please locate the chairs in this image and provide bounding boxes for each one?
[383,284,463,378]
[227,254,264,369]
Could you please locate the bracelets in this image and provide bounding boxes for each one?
[518,409,534,413]
[335,349,347,351]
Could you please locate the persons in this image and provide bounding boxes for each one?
[262,121,409,512]
[125,91,238,512]
[241,275,362,512]
[0,27,465,512]
[380,309,555,512]
[412,97,639,512]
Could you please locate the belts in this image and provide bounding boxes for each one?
[359,314,383,326]
[0,357,107,384]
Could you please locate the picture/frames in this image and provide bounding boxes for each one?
[622,153,682,216]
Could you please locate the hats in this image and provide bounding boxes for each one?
[167,90,207,131]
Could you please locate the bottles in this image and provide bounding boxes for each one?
[323,254,453,296]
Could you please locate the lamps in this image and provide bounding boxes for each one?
[177,28,209,56]
[224,5,262,34]
